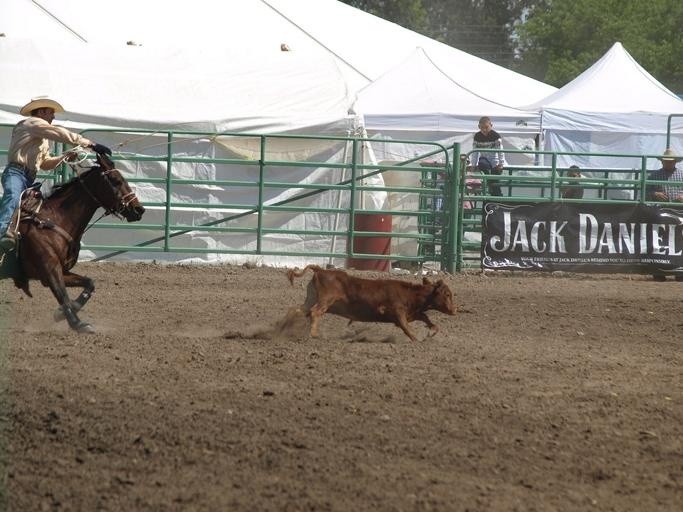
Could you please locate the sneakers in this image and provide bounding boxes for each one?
[0,233,17,252]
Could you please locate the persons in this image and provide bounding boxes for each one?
[556,165,582,199]
[642,148,683,283]
[470,116,505,197]
[0,94,98,251]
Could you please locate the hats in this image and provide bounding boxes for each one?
[20,95,65,115]
[657,149,683,161]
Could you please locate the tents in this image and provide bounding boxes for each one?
[512,40,683,202]
[0,2,557,266]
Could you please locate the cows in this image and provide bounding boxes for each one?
[286,264,458,342]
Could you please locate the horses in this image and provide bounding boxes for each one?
[0,153,145,334]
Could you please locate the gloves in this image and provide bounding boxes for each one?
[88,141,112,157]
